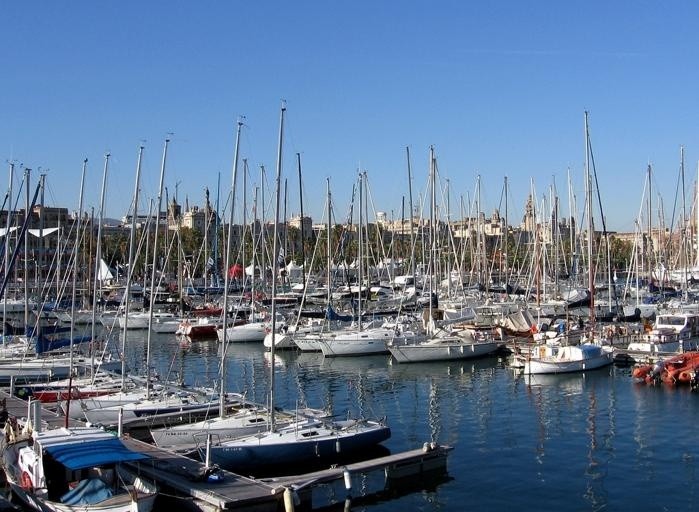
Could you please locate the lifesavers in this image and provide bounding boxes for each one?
[22,471,33,495]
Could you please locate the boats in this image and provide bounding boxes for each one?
[0,375,160,511]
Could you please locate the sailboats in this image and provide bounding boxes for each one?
[0,96,699,450]
[195,98,395,474]
